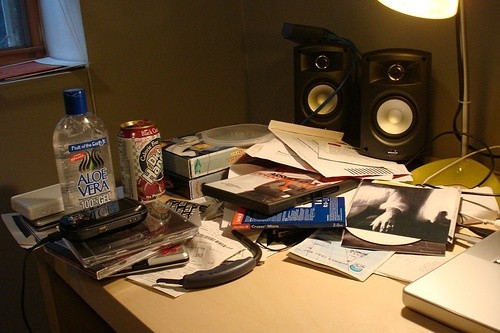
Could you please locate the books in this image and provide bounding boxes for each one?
[160,133,500,282]
[44,201,199,280]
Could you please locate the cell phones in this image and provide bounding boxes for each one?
[107,243,190,277]
[60,197,149,240]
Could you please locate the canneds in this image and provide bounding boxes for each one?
[117,119,166,201]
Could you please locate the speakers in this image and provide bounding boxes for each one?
[359,48,434,161]
[293,43,359,145]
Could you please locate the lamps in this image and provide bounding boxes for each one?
[376,0,500,224]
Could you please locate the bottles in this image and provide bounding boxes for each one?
[52,87,116,213]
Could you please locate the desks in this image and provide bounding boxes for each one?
[27,125,500,333]
